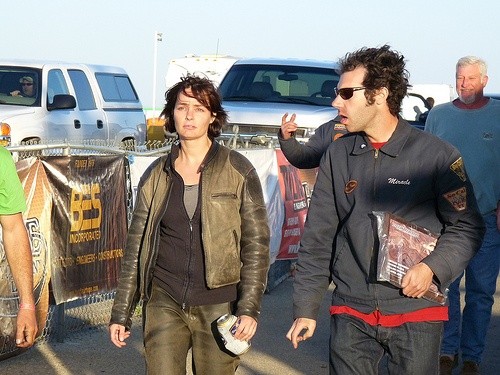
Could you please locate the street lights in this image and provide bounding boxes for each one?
[152,30,162,124]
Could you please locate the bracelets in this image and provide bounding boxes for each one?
[19,303,36,310]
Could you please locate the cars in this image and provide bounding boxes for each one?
[215,59,338,146]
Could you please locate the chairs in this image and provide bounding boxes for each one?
[237,82,281,97]
[310,80,339,100]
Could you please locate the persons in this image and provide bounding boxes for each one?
[10,77,35,97]
[419,97,434,121]
[278,113,349,169]
[285,44,487,374]
[424,56,500,375]
[0,147,38,348]
[109,71,271,375]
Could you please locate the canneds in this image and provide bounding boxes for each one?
[216,314,252,355]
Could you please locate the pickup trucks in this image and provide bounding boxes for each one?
[0,59,148,159]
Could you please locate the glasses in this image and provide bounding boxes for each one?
[334,87,370,100]
[22,82,33,86]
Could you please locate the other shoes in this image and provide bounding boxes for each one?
[461,361,480,375]
[438,350,459,375]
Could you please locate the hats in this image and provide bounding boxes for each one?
[20,77,34,83]
